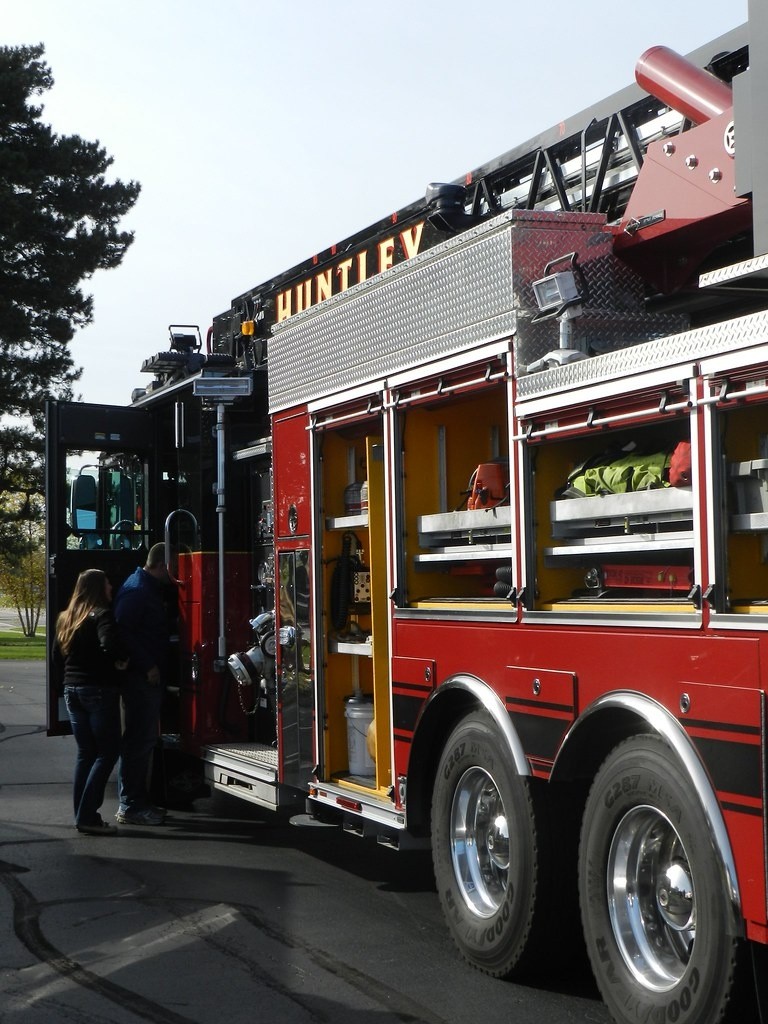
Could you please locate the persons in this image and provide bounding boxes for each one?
[51,569,130,834]
[112,542,174,826]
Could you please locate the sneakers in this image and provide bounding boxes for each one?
[117,806,167,826]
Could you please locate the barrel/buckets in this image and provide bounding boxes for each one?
[343,702,378,776]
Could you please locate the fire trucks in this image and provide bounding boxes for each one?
[43,16,768,1024]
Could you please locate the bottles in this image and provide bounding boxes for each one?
[359,480,369,515]
[345,486,360,515]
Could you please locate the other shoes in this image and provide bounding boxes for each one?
[76,819,118,835]
